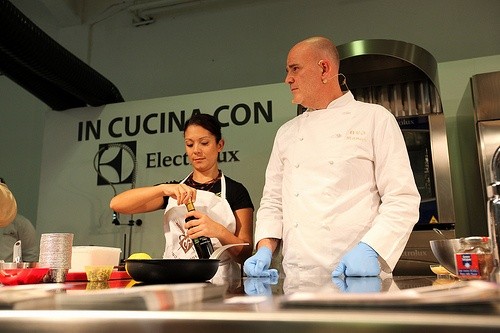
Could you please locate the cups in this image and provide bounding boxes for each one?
[0,263,18,270]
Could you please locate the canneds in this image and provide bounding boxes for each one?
[456,236,496,281]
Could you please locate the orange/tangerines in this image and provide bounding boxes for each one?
[125,252,152,276]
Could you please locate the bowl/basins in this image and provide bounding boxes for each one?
[67,246,123,272]
[38,233,74,283]
[86,282,109,291]
[84,265,115,282]
[0,268,50,285]
[429,236,494,276]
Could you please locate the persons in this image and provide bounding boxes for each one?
[242,36,422,277]
[0,177,40,263]
[110,114,255,285]
[136,277,400,311]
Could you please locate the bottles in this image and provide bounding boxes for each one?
[185,197,214,259]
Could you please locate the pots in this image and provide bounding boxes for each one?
[123,243,249,282]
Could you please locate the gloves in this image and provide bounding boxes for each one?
[242,247,279,277]
[332,242,380,277]
